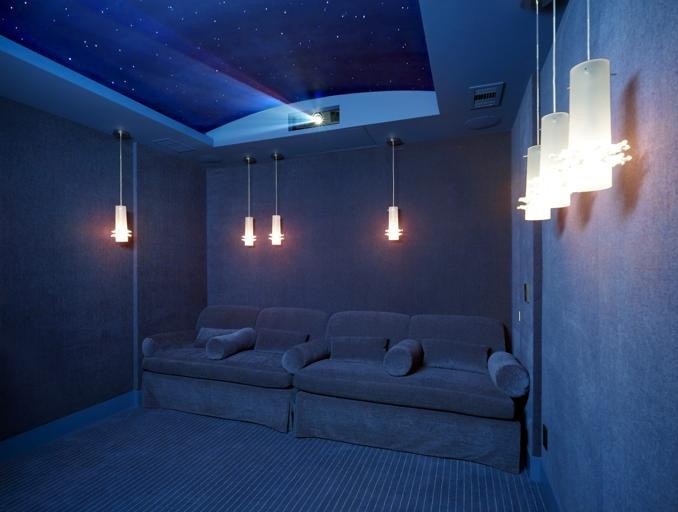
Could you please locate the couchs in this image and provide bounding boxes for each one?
[282,309,528,477]
[140,303,330,440]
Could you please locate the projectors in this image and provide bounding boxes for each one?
[293,109,339,129]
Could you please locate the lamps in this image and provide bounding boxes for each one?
[240,151,287,248]
[382,137,406,243]
[516,0,633,221]
[109,127,133,244]
